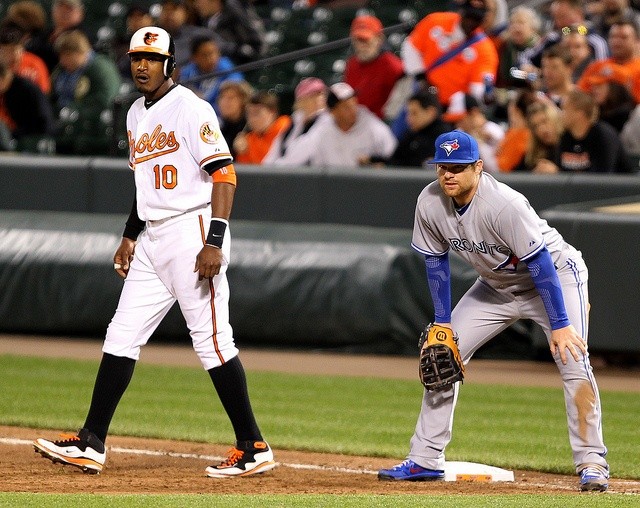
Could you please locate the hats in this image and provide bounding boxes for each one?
[344,14,385,45]
[426,129,479,164]
[441,91,477,120]
[125,26,175,56]
[329,81,359,106]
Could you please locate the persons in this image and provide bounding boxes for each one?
[377,129,611,490]
[0,0,140,158]
[141,2,215,104]
[33,26,280,479]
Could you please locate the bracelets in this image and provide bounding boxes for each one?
[205,217,229,248]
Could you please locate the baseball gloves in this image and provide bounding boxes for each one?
[417,324,464,393]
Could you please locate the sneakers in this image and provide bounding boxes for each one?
[32,427,107,474]
[580,466,608,490]
[377,458,445,481]
[203,440,276,478]
